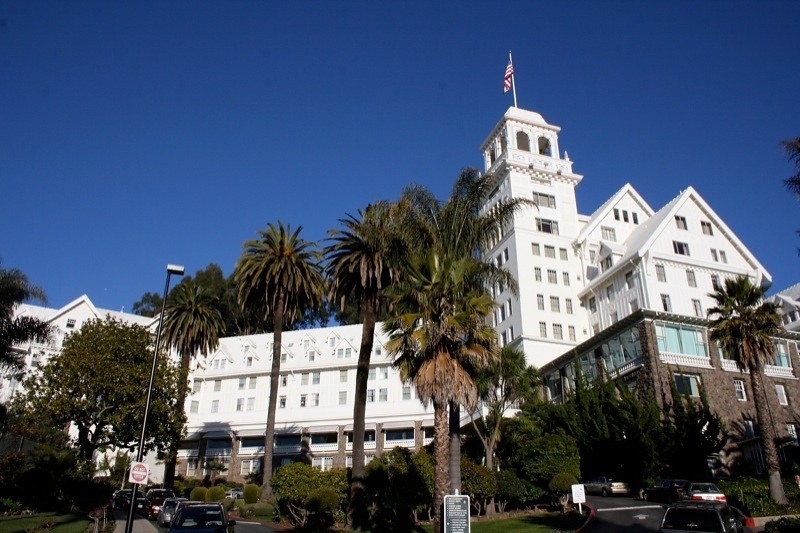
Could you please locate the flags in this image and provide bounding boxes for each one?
[503,60,514,94]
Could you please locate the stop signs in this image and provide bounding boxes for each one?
[127,461,149,486]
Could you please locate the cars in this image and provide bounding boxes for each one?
[113,489,235,533]
[223,487,243,499]
[648,479,755,533]
[583,477,628,497]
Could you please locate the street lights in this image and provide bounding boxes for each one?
[125,264,186,533]
